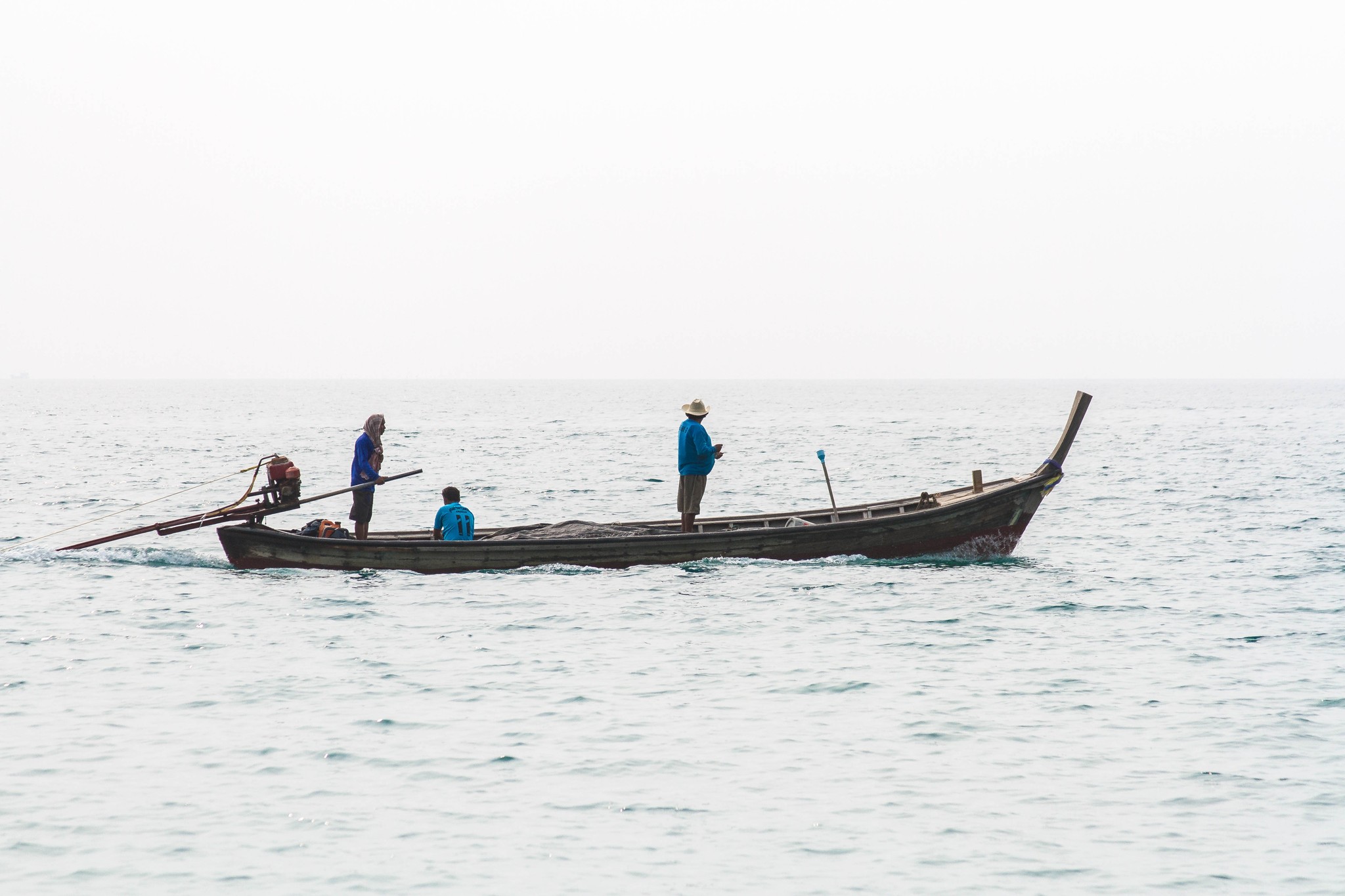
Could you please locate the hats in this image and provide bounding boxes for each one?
[682,399,710,416]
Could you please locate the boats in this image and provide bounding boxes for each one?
[216,389,1094,572]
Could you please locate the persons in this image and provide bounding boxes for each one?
[678,399,724,533]
[349,414,388,540]
[433,487,474,540]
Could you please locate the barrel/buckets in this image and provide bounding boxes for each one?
[785,517,816,527]
[318,520,341,538]
